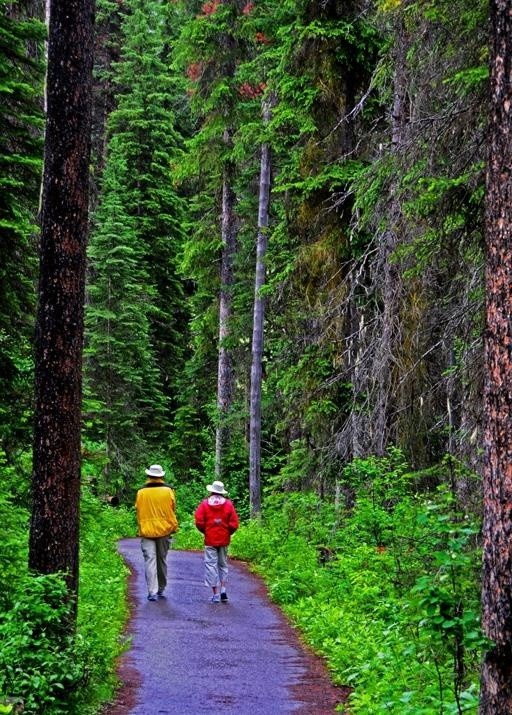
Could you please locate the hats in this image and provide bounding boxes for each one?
[145,464,166,478]
[206,480,228,494]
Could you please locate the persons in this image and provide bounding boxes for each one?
[135,464,178,601]
[195,480,238,603]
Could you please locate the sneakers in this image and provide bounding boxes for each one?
[156,591,164,597]
[147,594,157,600]
[220,592,229,601]
[212,594,220,601]
